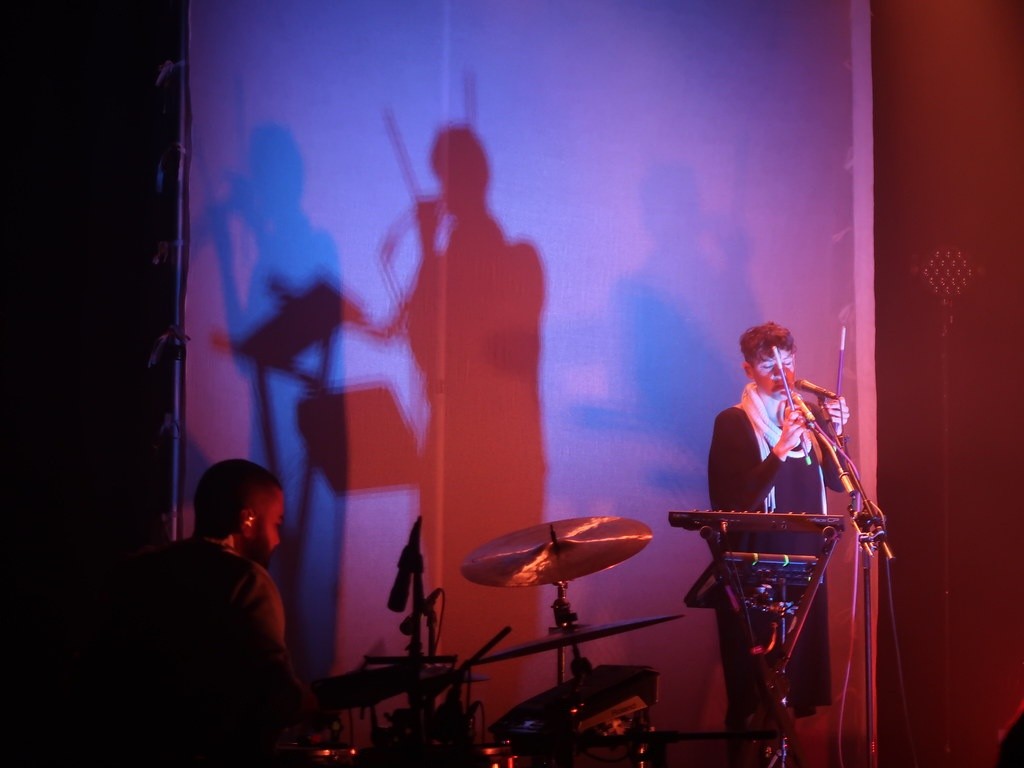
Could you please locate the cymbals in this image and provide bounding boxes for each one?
[457,514,655,588]
[464,609,688,671]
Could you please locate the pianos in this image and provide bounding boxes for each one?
[664,507,847,540]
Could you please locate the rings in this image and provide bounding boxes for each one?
[786,418,794,421]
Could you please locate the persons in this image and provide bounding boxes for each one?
[707,318,849,768]
[101,459,321,768]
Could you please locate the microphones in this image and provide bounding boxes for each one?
[790,391,816,423]
[399,589,442,636]
[467,702,480,718]
[795,378,839,400]
[387,518,423,613]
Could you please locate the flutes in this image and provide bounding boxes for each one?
[787,385,858,496]
